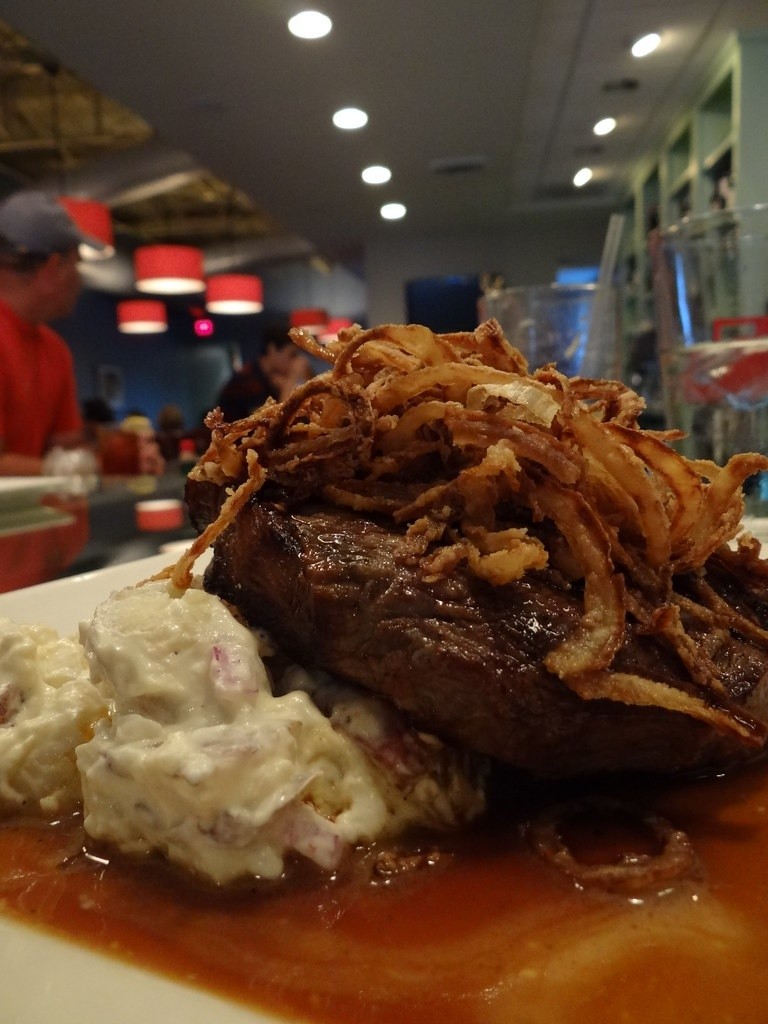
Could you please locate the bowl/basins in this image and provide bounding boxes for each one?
[137,500,184,532]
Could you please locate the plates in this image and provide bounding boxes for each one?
[0,547,768,1024]
[1,477,61,510]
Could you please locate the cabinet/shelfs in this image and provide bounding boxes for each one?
[619,32,768,506]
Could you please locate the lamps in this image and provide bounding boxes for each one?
[61,196,116,261]
[116,300,168,335]
[206,205,265,316]
[133,196,208,296]
[134,497,188,535]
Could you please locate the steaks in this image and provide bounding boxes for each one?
[178,470,768,780]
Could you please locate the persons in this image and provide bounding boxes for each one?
[117,408,164,475]
[297,358,317,382]
[0,191,100,477]
[204,308,304,459]
[82,371,119,426]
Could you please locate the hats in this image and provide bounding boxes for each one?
[0,190,114,261]
